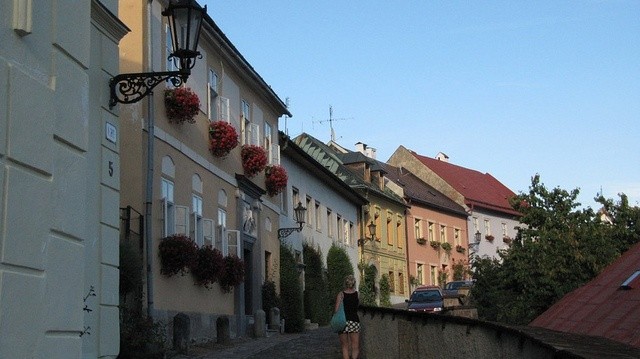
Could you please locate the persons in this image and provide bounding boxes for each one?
[335,275,362,359]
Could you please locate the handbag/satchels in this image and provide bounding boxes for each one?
[331,293,346,333]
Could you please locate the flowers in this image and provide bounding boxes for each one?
[208,120,239,159]
[265,164,289,198]
[163,84,201,125]
[242,143,268,181]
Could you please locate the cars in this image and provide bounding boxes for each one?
[404,285,445,314]
[442,279,475,298]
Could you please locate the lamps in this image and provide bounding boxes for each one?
[109,0,207,109]
[278,202,307,240]
[358,221,376,246]
[468,229,481,247]
